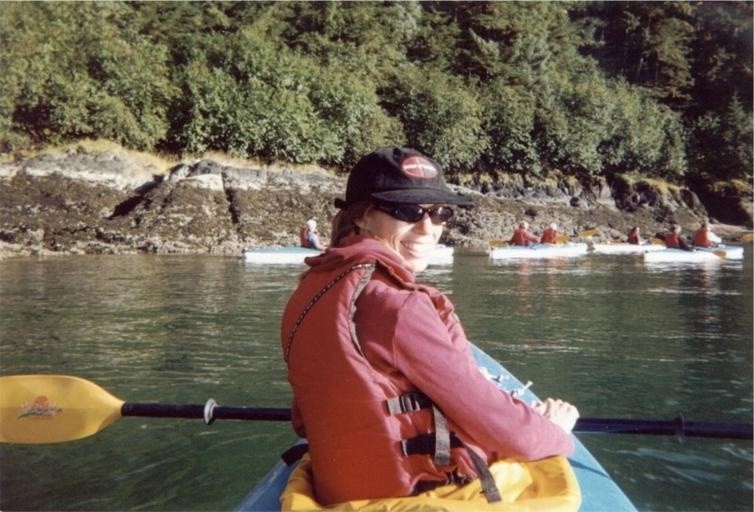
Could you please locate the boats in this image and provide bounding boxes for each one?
[591,241,667,255]
[242,244,457,266]
[232,337,639,512]
[642,243,745,263]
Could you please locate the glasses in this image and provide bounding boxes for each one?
[375,202,455,225]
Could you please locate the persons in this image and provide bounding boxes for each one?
[694,222,722,247]
[280,147,580,506]
[627,227,640,245]
[508,221,539,246]
[300,220,326,250]
[540,223,558,243]
[665,224,692,251]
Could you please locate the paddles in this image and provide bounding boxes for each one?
[611,233,754,257]
[489,228,598,246]
[0,373,753,445]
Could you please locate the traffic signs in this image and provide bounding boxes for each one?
[488,243,588,260]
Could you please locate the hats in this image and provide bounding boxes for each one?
[334,147,475,210]
